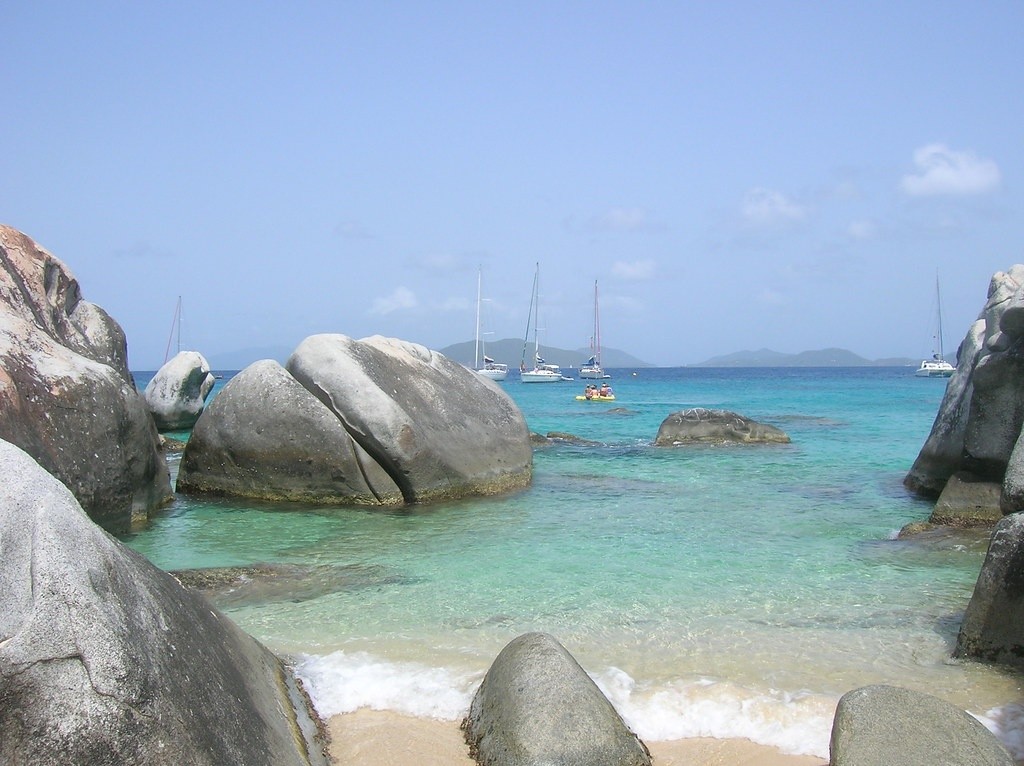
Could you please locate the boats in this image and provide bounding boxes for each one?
[632,372,637,376]
[214,374,223,379]
[575,394,615,400]
[603,373,610,378]
[562,374,574,381]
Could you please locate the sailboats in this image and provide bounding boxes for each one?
[520,262,562,382]
[916,266,957,377]
[580,280,604,379]
[475,263,510,380]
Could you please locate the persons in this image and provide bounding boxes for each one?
[600,383,613,397]
[585,384,598,399]
